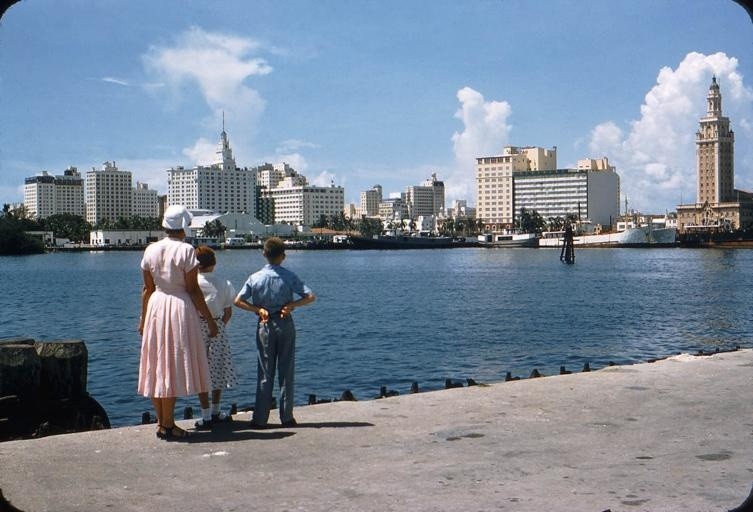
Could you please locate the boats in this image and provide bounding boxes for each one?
[476,231,535,248]
[347,231,453,248]
[537,195,678,247]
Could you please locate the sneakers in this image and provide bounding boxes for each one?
[250,419,264,428]
[195,412,232,427]
[283,418,297,426]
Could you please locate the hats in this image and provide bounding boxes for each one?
[196,247,216,268]
[162,205,193,237]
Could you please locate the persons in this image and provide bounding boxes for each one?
[194,245,236,430]
[136,203,218,440]
[234,236,317,428]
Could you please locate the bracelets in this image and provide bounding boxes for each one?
[140,315,145,322]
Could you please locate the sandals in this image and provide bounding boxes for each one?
[156,425,198,441]
